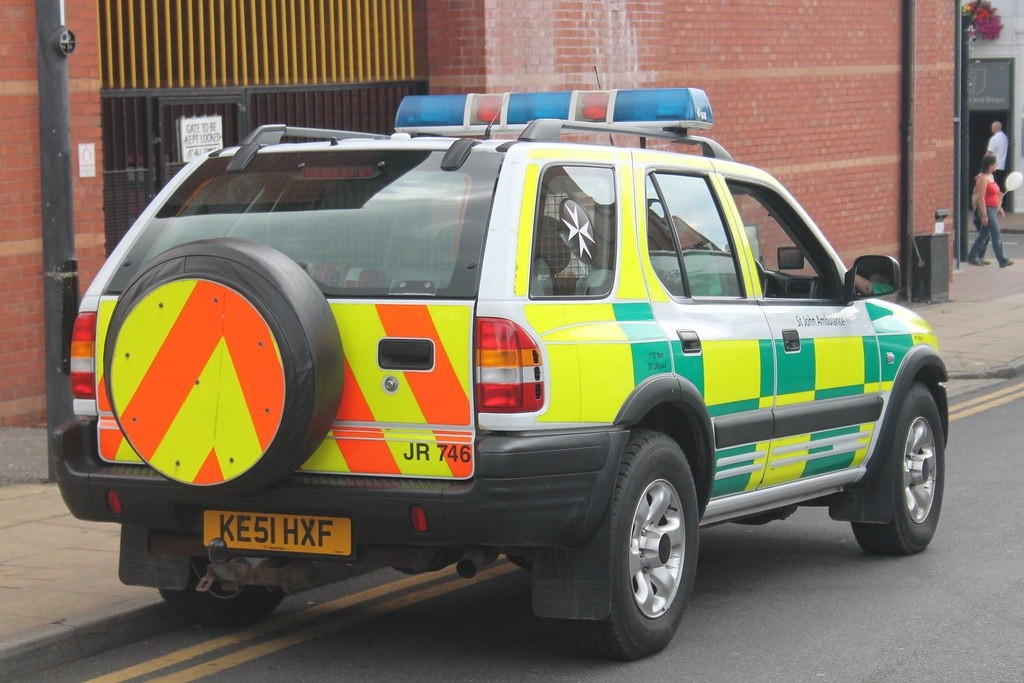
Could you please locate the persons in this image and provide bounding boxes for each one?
[967,121,1014,268]
[855,274,893,295]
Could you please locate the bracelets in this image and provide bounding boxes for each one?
[982,216,987,217]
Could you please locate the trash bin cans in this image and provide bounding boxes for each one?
[912,231,952,303]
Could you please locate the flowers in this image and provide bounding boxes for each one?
[961,0,1004,41]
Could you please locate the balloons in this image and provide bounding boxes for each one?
[1006,172,1023,191]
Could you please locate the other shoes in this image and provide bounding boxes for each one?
[1000,260,1014,268]
[968,255,990,266]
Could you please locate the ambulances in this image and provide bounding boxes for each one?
[49,89,949,663]
